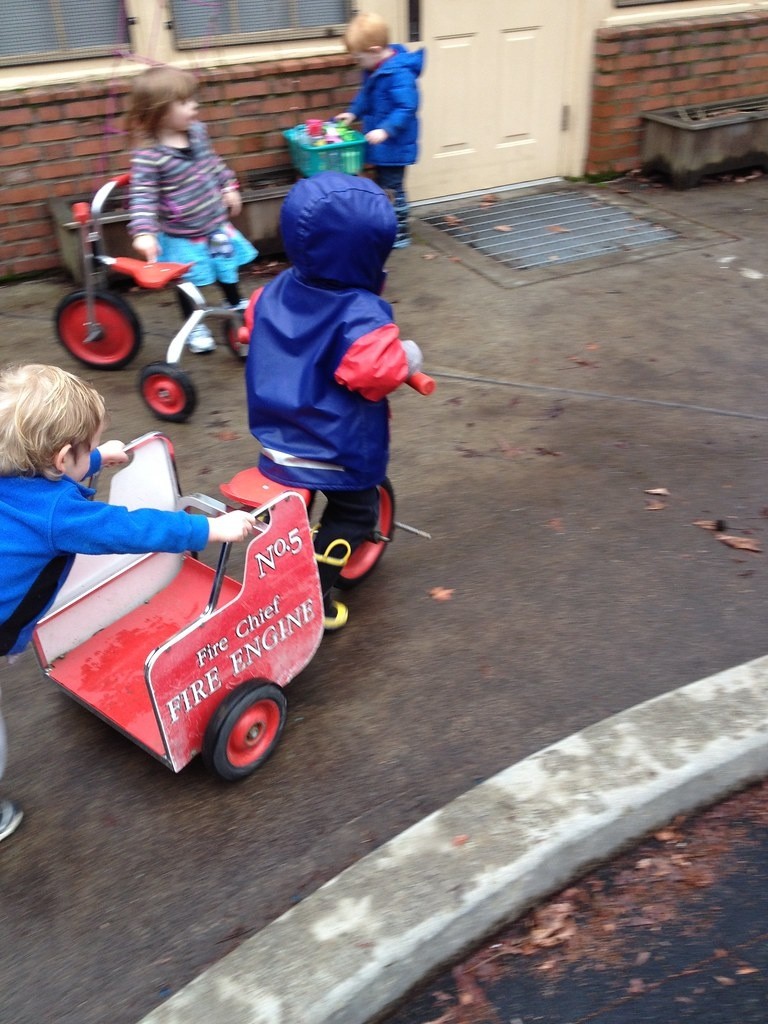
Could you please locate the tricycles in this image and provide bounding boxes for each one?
[58,172,253,423]
[27,328,436,783]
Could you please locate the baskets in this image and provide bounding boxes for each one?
[282,124,376,182]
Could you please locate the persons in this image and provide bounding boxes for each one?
[336,8,424,247]
[129,66,259,353]
[1,362,251,842]
[245,168,422,630]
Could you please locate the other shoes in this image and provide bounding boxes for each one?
[0,798,25,843]
[321,600,350,631]
[189,325,214,354]
[394,218,416,248]
